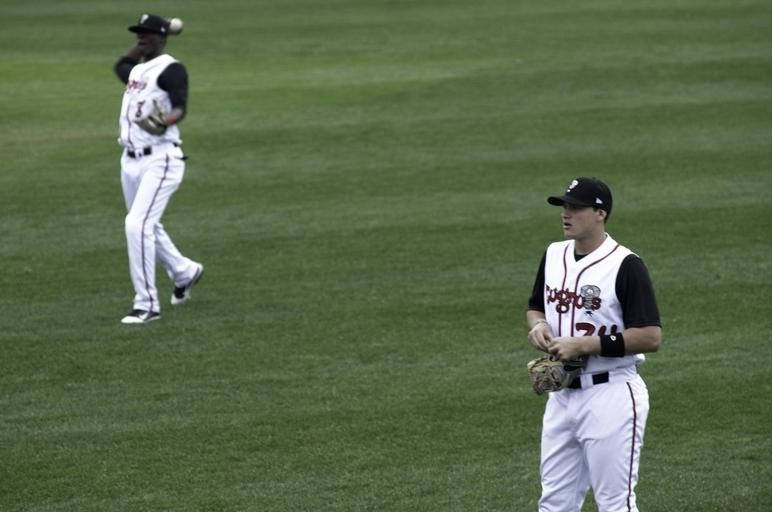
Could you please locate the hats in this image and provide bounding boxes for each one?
[129,14,171,38]
[548,177,612,213]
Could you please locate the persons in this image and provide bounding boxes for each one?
[525,177,662,512]
[114,12,204,324]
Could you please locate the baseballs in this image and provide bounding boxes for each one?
[170,18,182,33]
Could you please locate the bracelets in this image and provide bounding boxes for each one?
[601,332,625,358]
[165,114,175,125]
[534,320,547,324]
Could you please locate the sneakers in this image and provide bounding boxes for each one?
[170,264,204,304]
[121,308,162,324]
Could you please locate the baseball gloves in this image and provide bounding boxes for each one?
[527,354,586,396]
[132,99,167,136]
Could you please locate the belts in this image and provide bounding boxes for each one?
[567,371,609,390]
[126,145,153,158]
